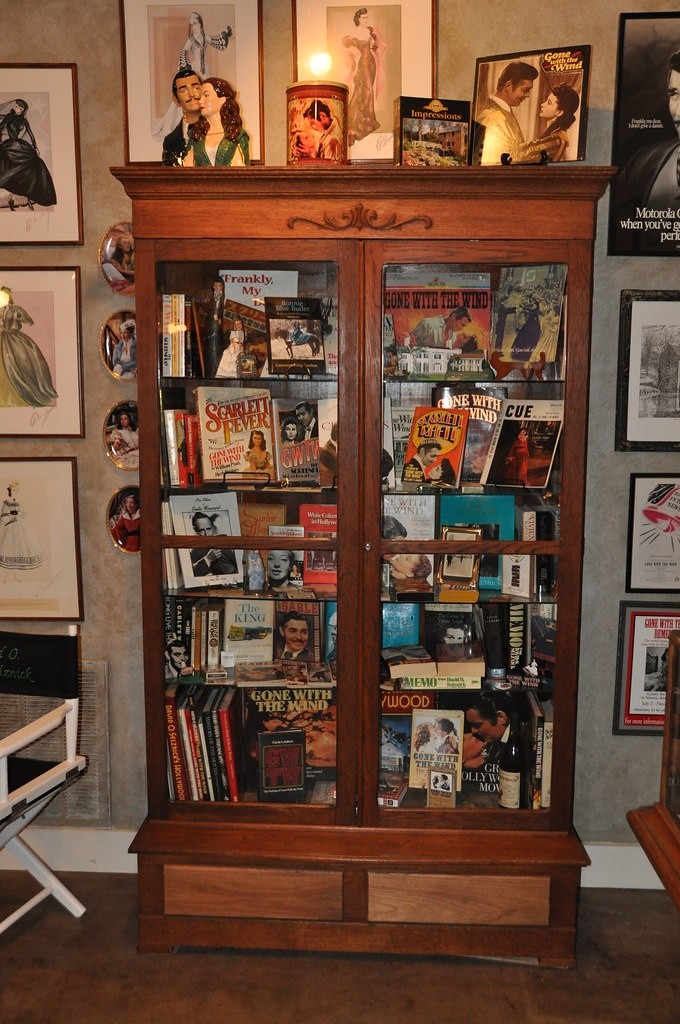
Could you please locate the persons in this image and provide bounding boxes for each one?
[204,276,226,376]
[495,284,560,363]
[413,717,458,754]
[433,718,459,753]
[462,733,500,793]
[161,68,250,167]
[267,550,302,588]
[190,512,239,577]
[216,319,245,378]
[112,319,136,378]
[381,516,432,592]
[319,422,338,485]
[291,100,344,161]
[241,429,275,479]
[475,61,579,167]
[617,48,680,201]
[466,692,534,767]
[280,401,319,446]
[101,236,135,286]
[114,495,141,552]
[401,439,457,486]
[110,411,139,468]
[163,639,191,679]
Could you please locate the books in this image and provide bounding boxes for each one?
[158,260,569,813]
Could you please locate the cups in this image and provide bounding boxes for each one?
[286,80,350,165]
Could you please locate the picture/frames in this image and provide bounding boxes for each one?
[616,286,680,453]
[609,600,680,738]
[0,60,86,246]
[0,267,86,439]
[625,472,680,593]
[291,2,437,162]
[0,457,85,622]
[465,43,589,165]
[122,0,263,164]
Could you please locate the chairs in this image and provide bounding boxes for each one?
[0,624,88,935]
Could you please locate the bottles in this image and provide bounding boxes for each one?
[499,712,528,810]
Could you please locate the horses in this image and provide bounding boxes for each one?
[274,327,320,358]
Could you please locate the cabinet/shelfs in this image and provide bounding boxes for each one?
[106,163,614,967]
